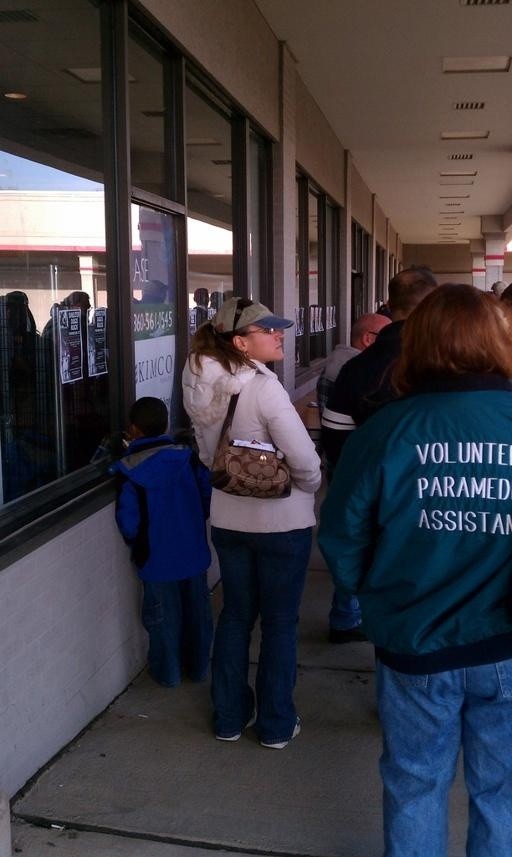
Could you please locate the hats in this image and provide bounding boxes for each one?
[210,297,294,333]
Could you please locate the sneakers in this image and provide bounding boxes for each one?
[214,707,301,749]
[330,626,368,643]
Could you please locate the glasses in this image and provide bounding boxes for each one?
[250,327,275,334]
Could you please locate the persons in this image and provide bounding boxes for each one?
[315,272,438,642]
[108,397,214,687]
[183,298,322,749]
[490,281,512,305]
[318,280,510,857]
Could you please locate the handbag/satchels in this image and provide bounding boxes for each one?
[213,430,291,499]
